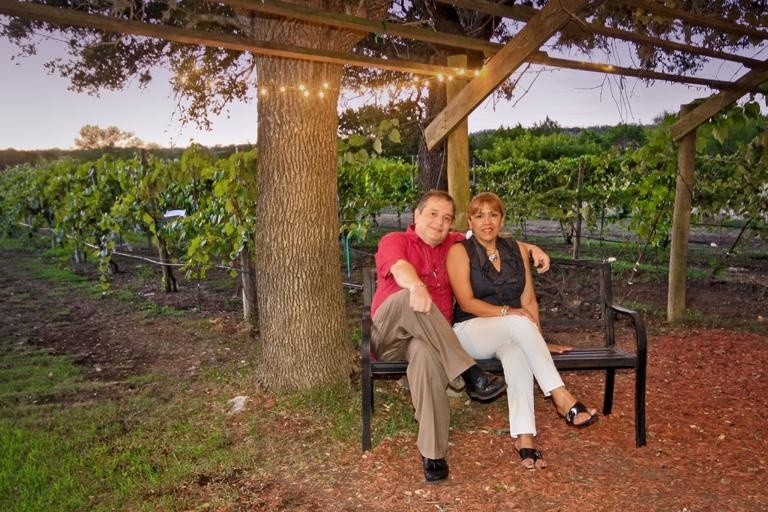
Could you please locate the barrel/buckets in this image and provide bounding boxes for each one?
[557,402,599,430]
[513,445,546,470]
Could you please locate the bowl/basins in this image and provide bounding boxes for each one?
[486,249,498,261]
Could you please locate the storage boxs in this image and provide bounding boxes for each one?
[361,258,648,451]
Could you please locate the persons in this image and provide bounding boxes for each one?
[443,189,601,472]
[368,189,553,483]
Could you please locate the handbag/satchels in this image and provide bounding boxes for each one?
[408,283,428,291]
[500,304,509,317]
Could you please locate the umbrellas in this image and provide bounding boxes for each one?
[466,372,508,405]
[423,456,449,482]
